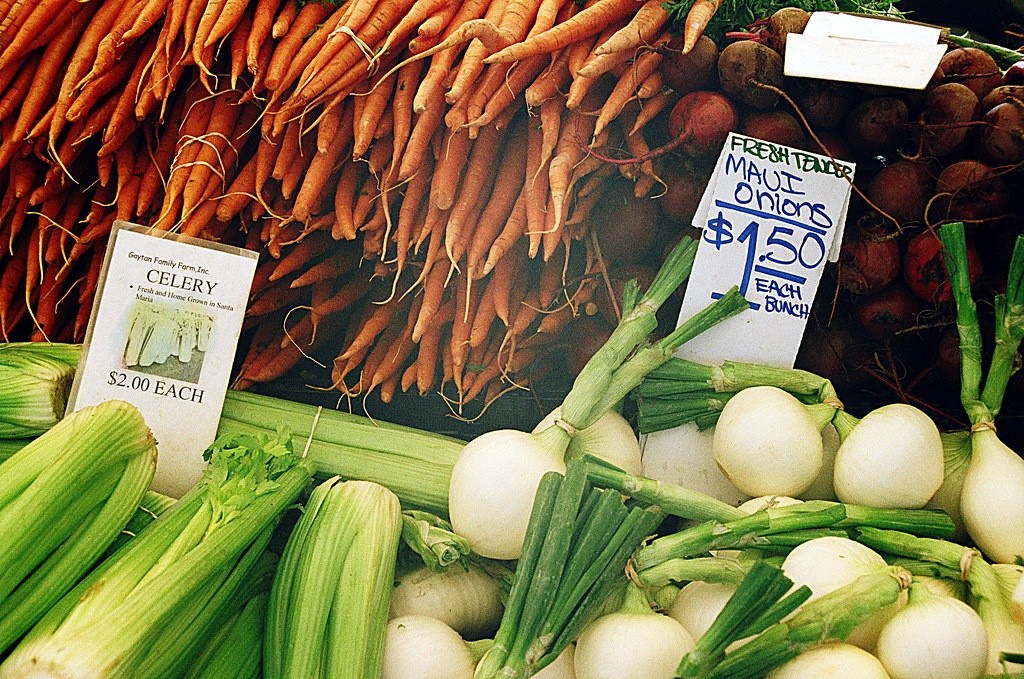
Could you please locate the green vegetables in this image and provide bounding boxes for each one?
[0,340,480,679]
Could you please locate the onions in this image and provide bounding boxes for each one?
[380,401,1024,679]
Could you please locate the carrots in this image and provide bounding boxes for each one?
[0,0,721,411]
